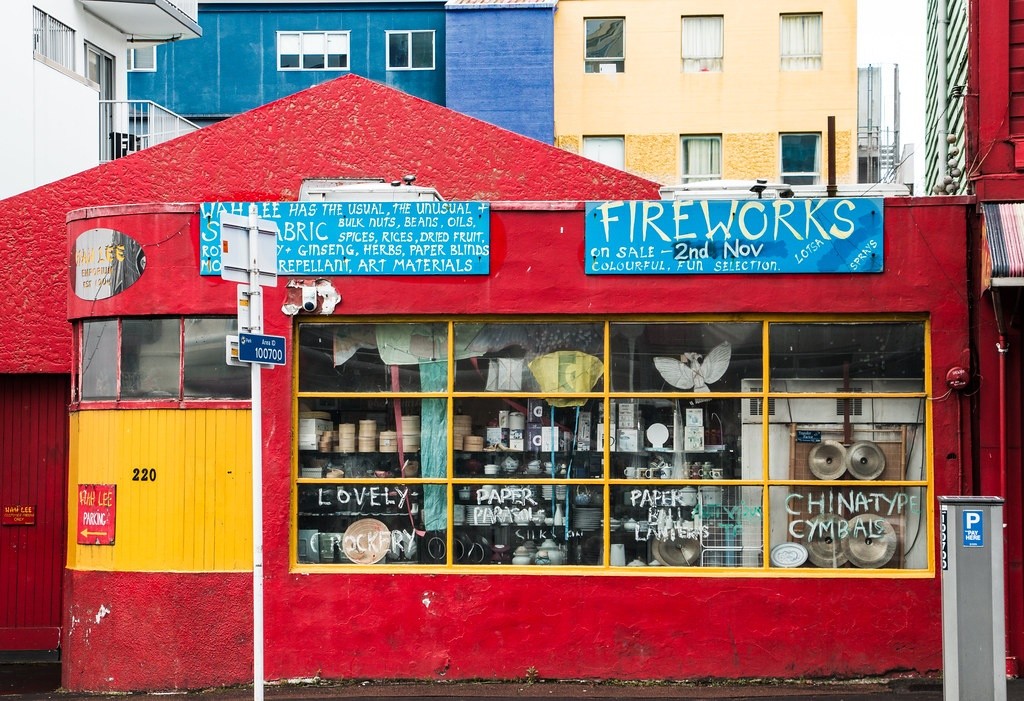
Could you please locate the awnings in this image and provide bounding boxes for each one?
[980,204,1024,301]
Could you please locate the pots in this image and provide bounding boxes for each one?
[509,412,525,429]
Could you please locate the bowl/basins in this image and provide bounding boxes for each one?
[420,532,447,564]
[531,515,544,526]
[470,536,491,563]
[544,517,554,526]
[453,532,473,564]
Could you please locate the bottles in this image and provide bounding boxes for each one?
[554,504,563,525]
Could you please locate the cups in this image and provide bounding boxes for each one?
[645,466,672,479]
[601,517,648,534]
[683,462,723,479]
[679,486,696,507]
[514,512,529,527]
[635,468,647,479]
[498,510,512,526]
[484,460,552,474]
[624,467,635,478]
[452,505,465,525]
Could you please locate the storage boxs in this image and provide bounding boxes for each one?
[684,408,704,449]
[298,419,335,450]
[526,397,640,451]
[487,428,508,446]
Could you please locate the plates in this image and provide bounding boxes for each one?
[466,505,493,526]
[344,518,391,564]
[572,508,601,530]
[770,543,807,567]
[539,485,569,501]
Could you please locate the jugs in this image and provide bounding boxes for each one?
[610,543,626,567]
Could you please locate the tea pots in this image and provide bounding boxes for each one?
[573,485,591,507]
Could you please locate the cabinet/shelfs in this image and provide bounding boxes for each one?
[297,394,735,567]
